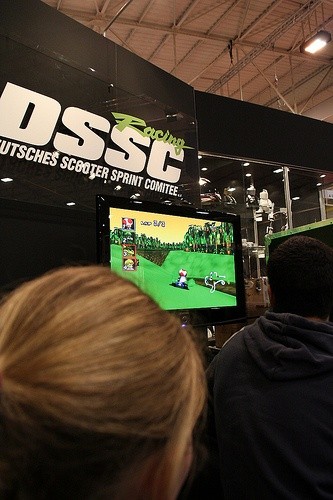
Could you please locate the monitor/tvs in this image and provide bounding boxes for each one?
[96,193,248,326]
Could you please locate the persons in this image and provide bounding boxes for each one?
[204,235,333,500]
[1,265,208,499]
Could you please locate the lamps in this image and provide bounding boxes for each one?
[299,29,332,56]
